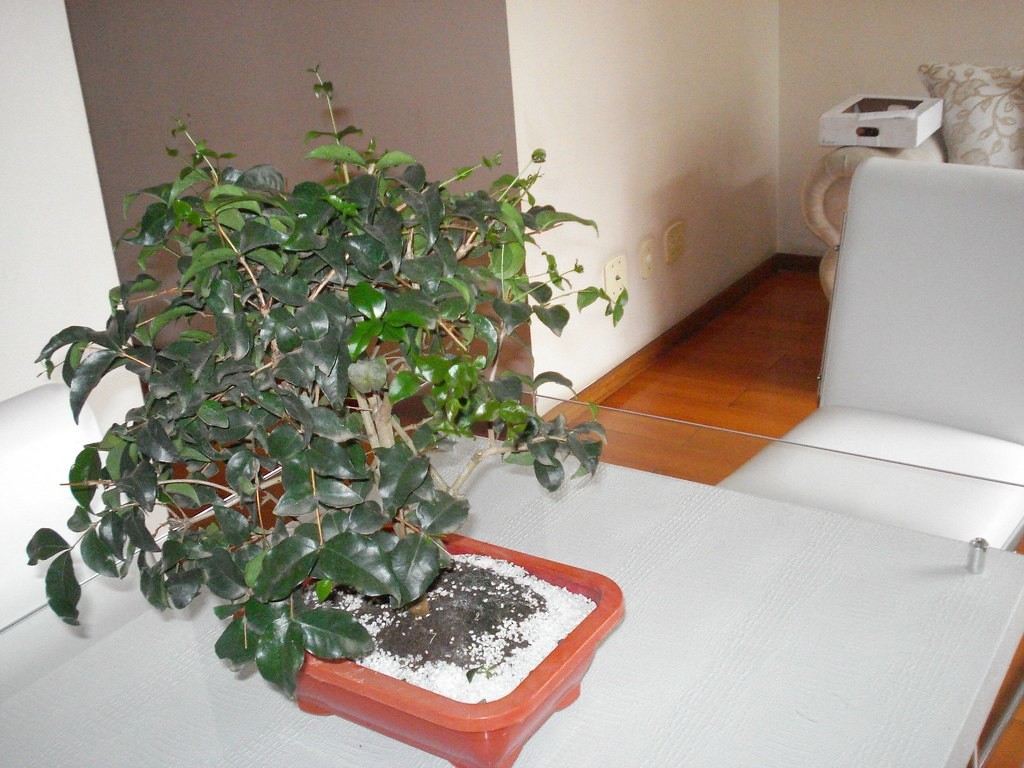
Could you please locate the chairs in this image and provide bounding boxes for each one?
[1,381,129,634]
[713,159,1024,768]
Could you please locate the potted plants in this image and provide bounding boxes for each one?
[27,62,628,768]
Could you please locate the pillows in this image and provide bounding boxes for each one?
[920,63,1023,170]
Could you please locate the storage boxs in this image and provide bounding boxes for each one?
[818,93,943,148]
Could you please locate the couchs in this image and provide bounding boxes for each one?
[801,134,946,302]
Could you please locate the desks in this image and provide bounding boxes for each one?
[0,434,1024,768]
